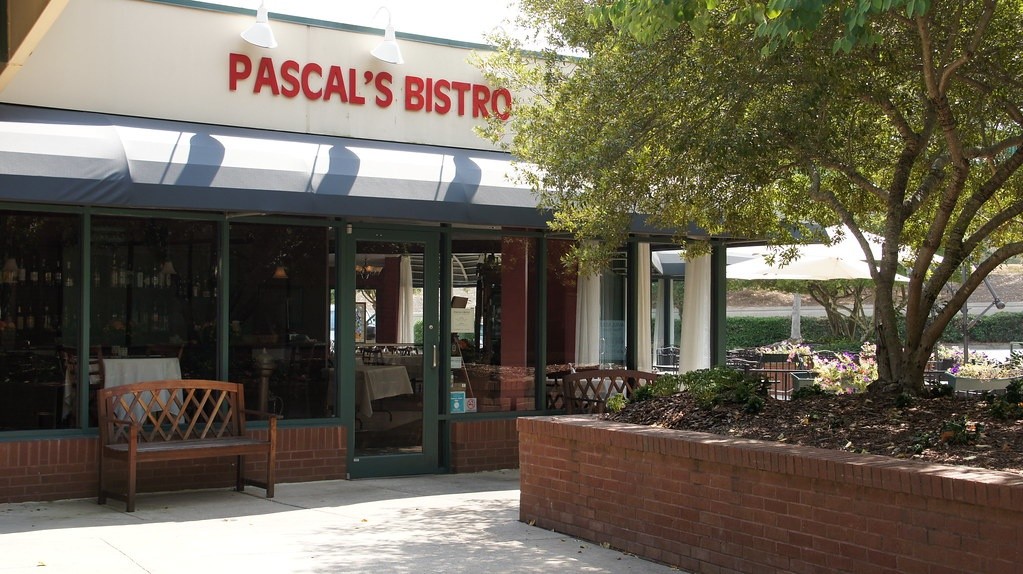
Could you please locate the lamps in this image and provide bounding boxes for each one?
[240,0,278,49]
[271,264,289,279]
[371,5,404,65]
[356,258,384,279]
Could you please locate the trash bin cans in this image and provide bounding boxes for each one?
[791,371,820,391]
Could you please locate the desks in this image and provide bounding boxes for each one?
[318,365,414,429]
[52,355,184,427]
[363,353,423,399]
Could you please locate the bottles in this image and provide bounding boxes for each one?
[0,301,171,333]
[0,252,218,297]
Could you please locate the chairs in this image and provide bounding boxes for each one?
[660,346,762,369]
[61,343,106,428]
[146,342,186,364]
[546,372,570,410]
[246,340,316,420]
[355,347,420,400]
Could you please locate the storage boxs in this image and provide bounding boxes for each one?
[450,375,466,414]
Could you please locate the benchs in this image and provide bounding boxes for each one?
[563,370,662,416]
[97,380,276,511]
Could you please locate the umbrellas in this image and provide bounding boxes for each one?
[684,221,945,338]
